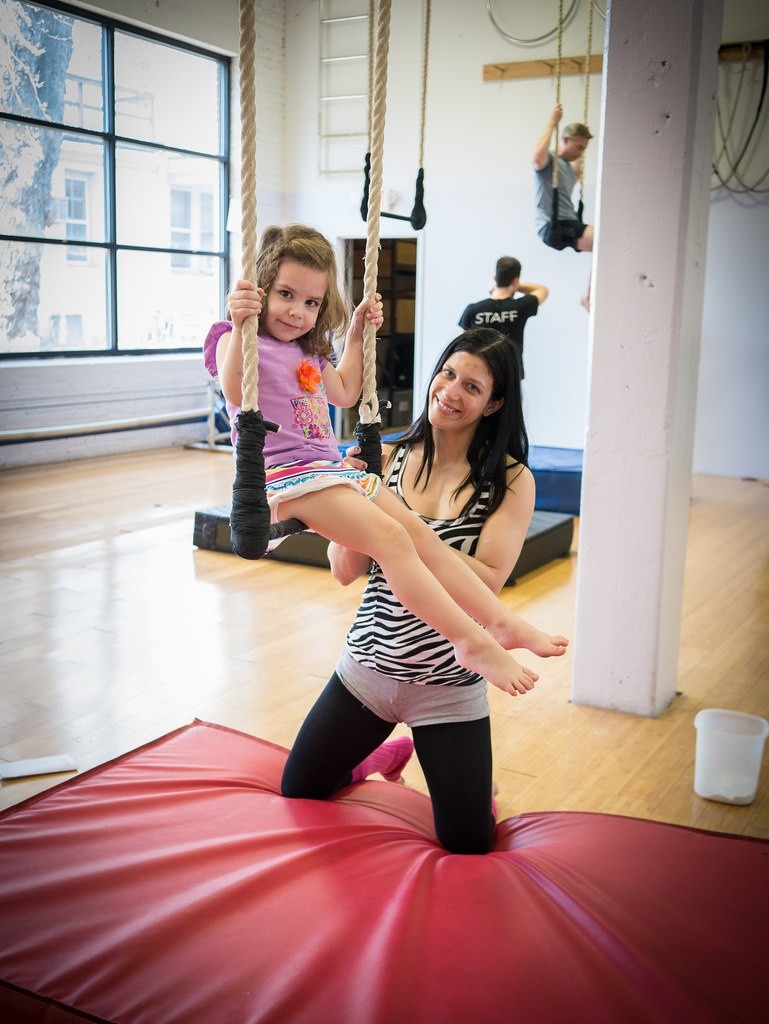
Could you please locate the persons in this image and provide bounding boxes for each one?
[457,257,547,379]
[532,103,594,314]
[279,328,536,856]
[203,224,568,698]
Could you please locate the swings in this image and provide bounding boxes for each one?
[551,0,595,247]
[230,1,393,560]
[360,0,432,230]
